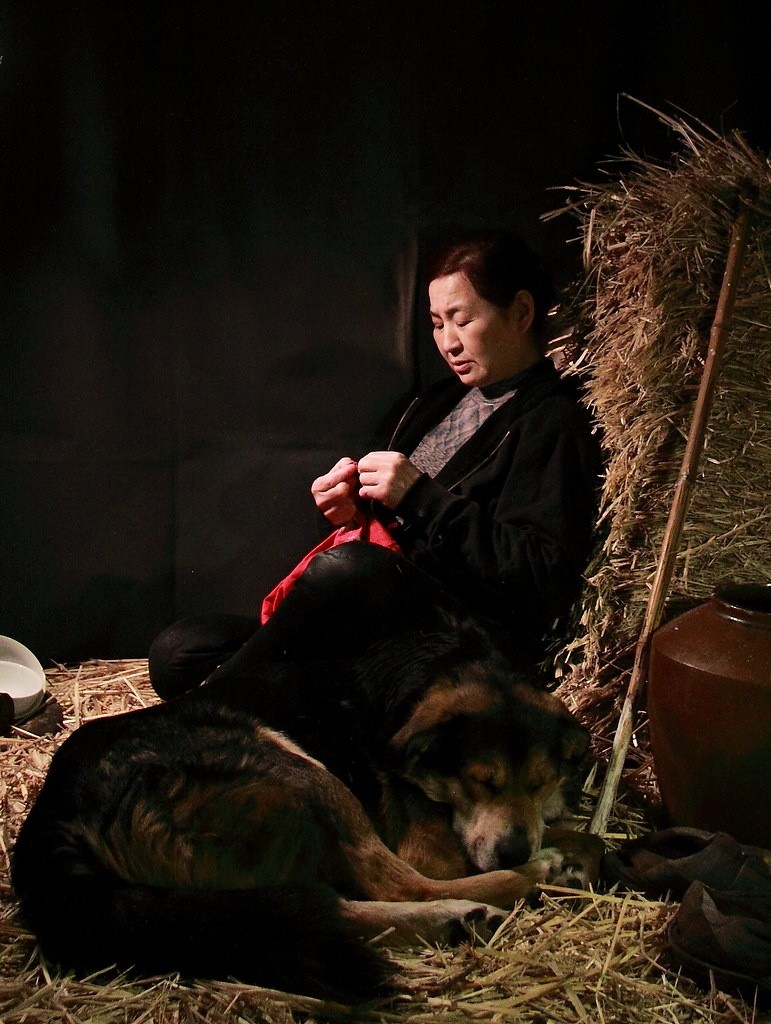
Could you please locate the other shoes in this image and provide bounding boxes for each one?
[666,879,771,1012]
[597,826,771,909]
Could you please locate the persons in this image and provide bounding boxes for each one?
[149,237,603,704]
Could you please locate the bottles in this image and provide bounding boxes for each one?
[651,582,771,846]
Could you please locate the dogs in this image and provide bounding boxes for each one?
[9,626,606,1008]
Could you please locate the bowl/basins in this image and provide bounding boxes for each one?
[0,634,46,720]
